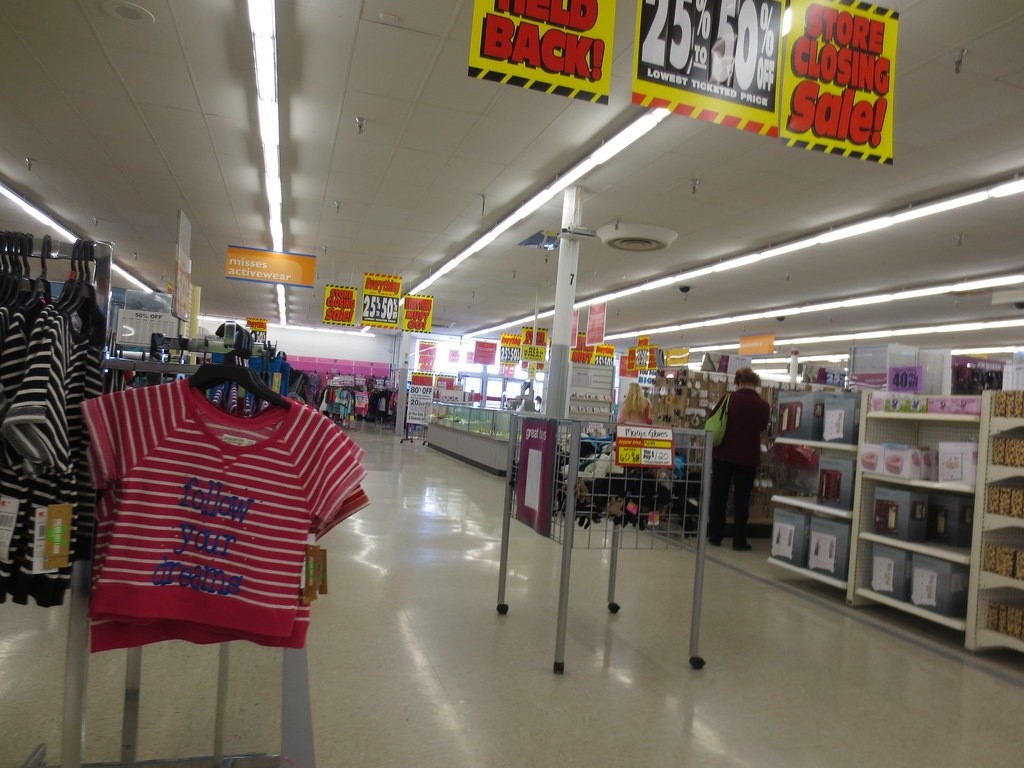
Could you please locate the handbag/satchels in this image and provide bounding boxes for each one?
[701,392,731,447]
[644,399,652,425]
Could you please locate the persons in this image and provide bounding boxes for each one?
[615,383,653,425]
[707,368,770,550]
[534,396,541,411]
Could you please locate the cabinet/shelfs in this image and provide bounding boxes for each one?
[426,402,540,477]
[764,389,1024,654]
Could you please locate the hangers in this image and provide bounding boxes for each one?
[0,230,108,336]
[113,318,342,441]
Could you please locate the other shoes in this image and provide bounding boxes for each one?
[733,543,752,551]
[708,538,720,547]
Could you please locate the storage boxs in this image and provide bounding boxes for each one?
[770,344,1024,640]
